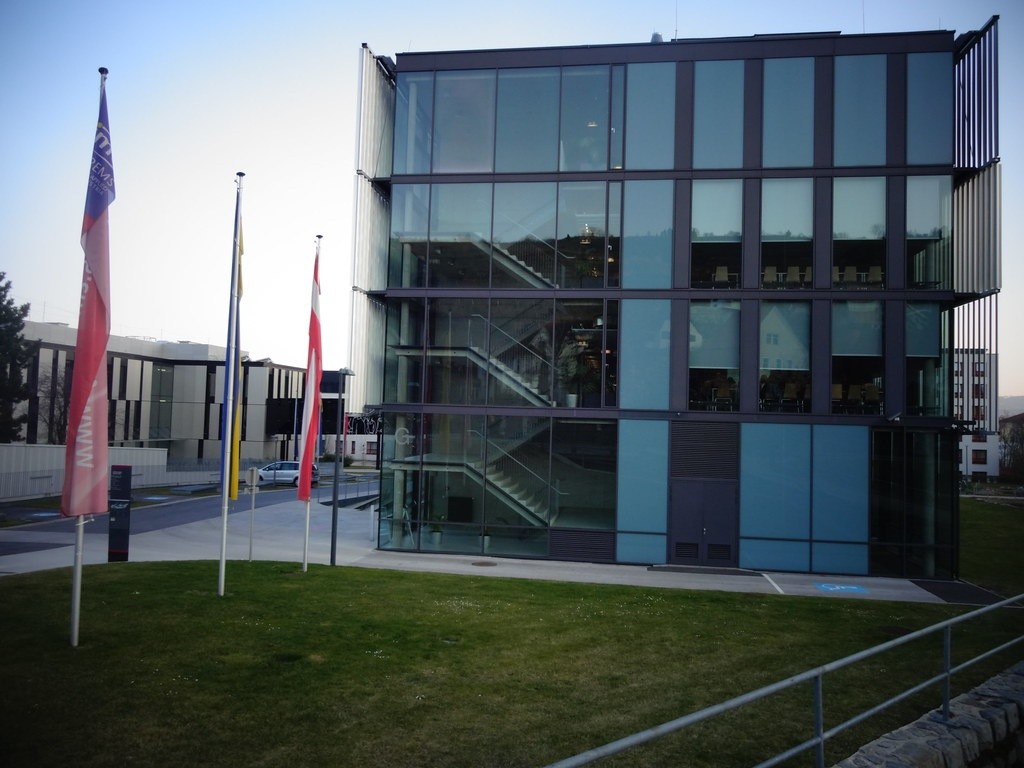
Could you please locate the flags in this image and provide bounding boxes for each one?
[219,189,243,502]
[342,405,349,459]
[61,84,117,519]
[297,248,323,502]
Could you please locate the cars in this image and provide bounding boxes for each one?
[258,460,320,488]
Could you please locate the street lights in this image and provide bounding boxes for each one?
[331,369,355,566]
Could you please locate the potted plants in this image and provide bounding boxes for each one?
[429,514,445,546]
[559,354,591,407]
[480,520,491,549]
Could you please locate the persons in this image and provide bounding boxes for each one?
[703,367,786,410]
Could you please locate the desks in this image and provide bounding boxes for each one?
[842,390,884,413]
[839,272,884,289]
[711,387,738,411]
[711,273,741,290]
[761,271,806,290]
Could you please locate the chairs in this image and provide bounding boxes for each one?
[763,263,886,290]
[693,381,885,414]
[714,265,730,290]
